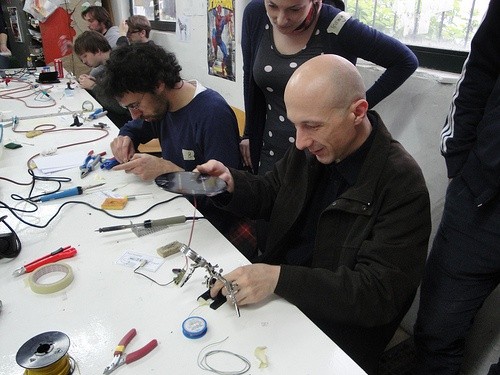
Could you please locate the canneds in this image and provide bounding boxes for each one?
[54,58,64,79]
[27,54,35,70]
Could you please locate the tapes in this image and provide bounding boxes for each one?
[29,261,75,294]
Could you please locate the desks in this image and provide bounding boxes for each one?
[0,66,368,375]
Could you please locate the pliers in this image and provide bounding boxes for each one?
[89,109,108,119]
[102,328,157,375]
[79,150,106,178]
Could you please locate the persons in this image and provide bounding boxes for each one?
[0,4,16,56]
[82,6,130,79]
[239,0,418,176]
[103,40,247,233]
[125,14,154,47]
[414,1,500,375]
[191,54,433,374]
[74,30,132,129]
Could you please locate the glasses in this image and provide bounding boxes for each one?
[127,30,141,35]
[124,92,146,112]
